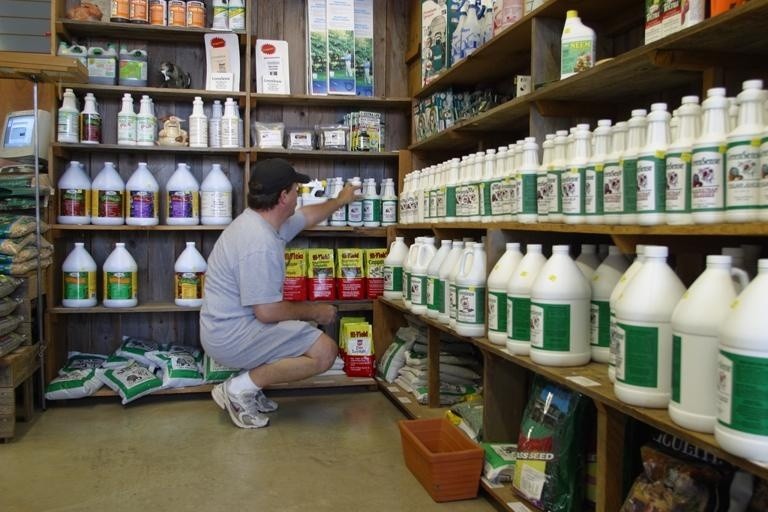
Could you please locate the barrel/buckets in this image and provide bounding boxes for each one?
[454,243,486,337]
[574,245,600,280]
[507,244,547,356]
[739,243,760,276]
[56,42,87,67]
[529,245,591,367]
[590,246,629,363]
[613,247,688,406]
[463,238,472,248]
[85,44,118,85]
[481,236,486,250]
[438,241,463,324]
[426,239,452,319]
[210,0,229,31]
[117,44,148,87]
[598,244,608,263]
[404,237,425,308]
[383,237,409,300]
[667,254,751,435]
[411,238,438,316]
[712,259,768,466]
[721,247,746,284]
[449,242,476,328]
[486,243,524,346]
[229,0,246,30]
[402,248,408,302]
[608,245,647,384]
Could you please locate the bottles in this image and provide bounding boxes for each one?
[188,94,245,147]
[399,136,540,225]
[327,177,397,227]
[381,236,487,338]
[136,95,158,146]
[165,162,199,226]
[116,93,136,146]
[111,0,208,30]
[173,242,206,307]
[201,164,232,225]
[125,162,159,225]
[58,160,92,224]
[58,88,80,143]
[80,93,101,144]
[446,0,521,67]
[61,243,97,308]
[561,10,597,80]
[91,162,126,225]
[103,243,138,307]
[536,79,768,226]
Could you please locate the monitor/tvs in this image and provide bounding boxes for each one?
[0,108,52,174]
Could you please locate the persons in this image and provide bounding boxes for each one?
[199,158,365,429]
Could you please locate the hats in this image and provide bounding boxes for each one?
[249,158,309,194]
[211,373,281,429]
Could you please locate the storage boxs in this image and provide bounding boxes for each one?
[405,44,421,94]
[411,0,422,50]
[397,418,485,503]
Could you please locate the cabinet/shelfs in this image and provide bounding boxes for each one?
[42,0,410,410]
[374,3,768,512]
